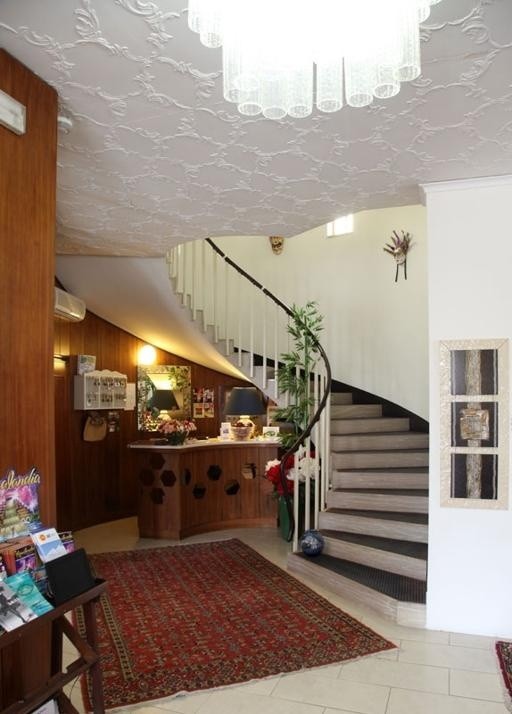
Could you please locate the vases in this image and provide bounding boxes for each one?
[278,498,295,541]
[166,431,187,445]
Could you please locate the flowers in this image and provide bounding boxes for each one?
[263,448,320,502]
[156,419,197,436]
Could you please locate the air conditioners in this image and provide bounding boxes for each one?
[53,286,86,322]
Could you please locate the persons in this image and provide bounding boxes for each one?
[0,586,29,625]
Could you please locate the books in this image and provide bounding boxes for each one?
[28,524,68,564]
[0,570,55,632]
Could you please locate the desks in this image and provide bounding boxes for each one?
[131,439,285,540]
[0,578,110,714]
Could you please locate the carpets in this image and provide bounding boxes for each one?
[70,537,399,714]
[490,640,512,714]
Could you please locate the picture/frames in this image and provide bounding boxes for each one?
[439,338,510,511]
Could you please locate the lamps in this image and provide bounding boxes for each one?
[187,0,441,120]
[269,235,285,255]
[223,386,267,438]
[139,344,156,366]
